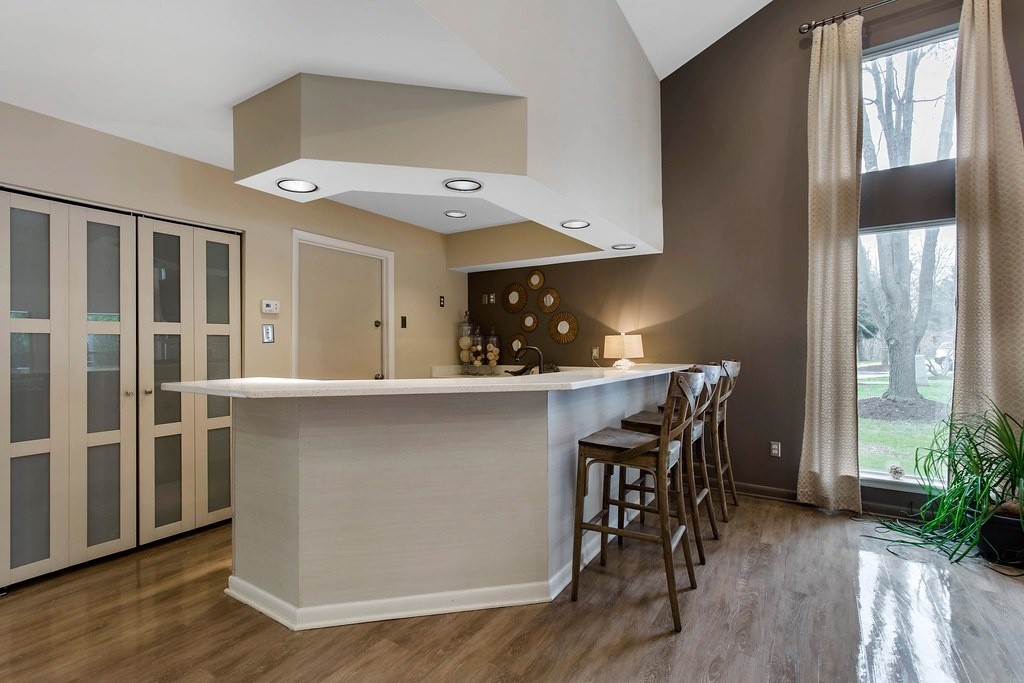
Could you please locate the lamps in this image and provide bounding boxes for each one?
[592,346,599,359]
[604,332,644,367]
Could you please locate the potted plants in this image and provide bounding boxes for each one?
[858,390,1024,573]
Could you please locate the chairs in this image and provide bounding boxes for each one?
[571,358,742,632]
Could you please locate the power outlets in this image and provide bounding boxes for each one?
[439,296,444,307]
[262,324,274,343]
[770,441,781,457]
[490,293,496,304]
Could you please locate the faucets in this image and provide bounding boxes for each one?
[513,346,544,374]
